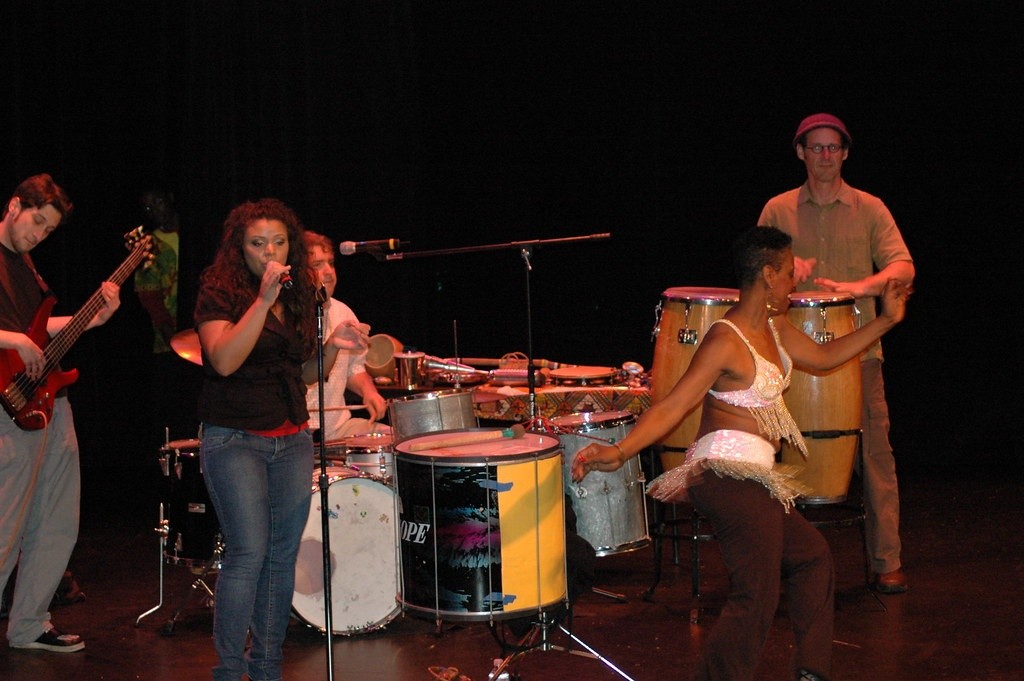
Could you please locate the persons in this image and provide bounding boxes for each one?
[299,231,400,451]
[133,175,186,361]
[0,175,120,654]
[569,227,910,681]
[755,112,916,593]
[189,199,371,681]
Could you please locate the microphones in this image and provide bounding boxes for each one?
[279,273,293,289]
[339,238,405,255]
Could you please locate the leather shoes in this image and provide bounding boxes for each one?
[12,620,85,652]
[877,570,906,592]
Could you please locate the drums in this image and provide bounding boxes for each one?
[390,425,568,622]
[312,431,397,478]
[160,439,225,571]
[290,466,403,635]
[649,287,741,503]
[777,290,864,504]
[387,388,477,440]
[548,366,621,385]
[547,409,651,556]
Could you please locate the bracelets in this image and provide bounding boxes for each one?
[613,444,625,461]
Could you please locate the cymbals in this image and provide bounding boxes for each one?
[171,328,203,367]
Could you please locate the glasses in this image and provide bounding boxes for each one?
[804,143,843,154]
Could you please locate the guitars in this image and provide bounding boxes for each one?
[0,226,155,432]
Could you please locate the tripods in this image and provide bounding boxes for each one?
[487,609,637,681]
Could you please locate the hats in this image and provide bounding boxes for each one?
[793,114,851,147]
